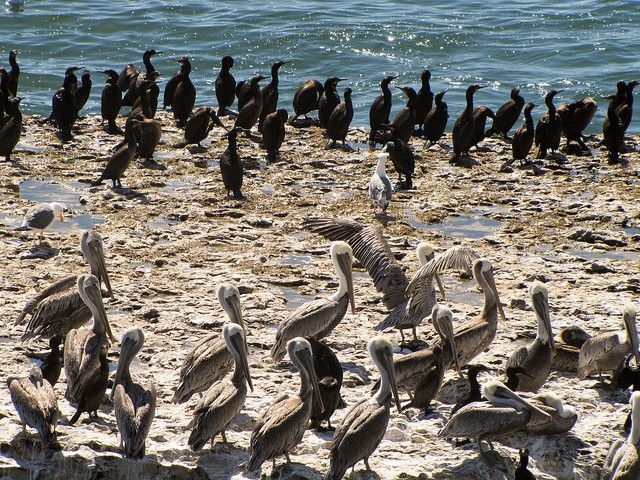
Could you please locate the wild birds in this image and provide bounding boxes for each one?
[599,79,640,148]
[617,80,627,103]
[560,97,599,151]
[15,202,64,250]
[290,79,324,125]
[370,153,393,215]
[601,391,640,480]
[245,338,326,480]
[307,335,344,433]
[113,80,163,152]
[271,241,356,364]
[186,106,230,148]
[8,49,20,97]
[299,216,484,342]
[513,102,540,167]
[132,71,165,109]
[264,109,289,160]
[1,96,25,162]
[52,66,86,138]
[215,56,237,115]
[221,126,251,201]
[0,68,16,119]
[390,85,418,144]
[425,88,450,144]
[173,58,197,123]
[381,122,416,189]
[98,69,122,126]
[536,89,565,158]
[40,69,92,125]
[580,303,640,392]
[63,273,116,424]
[6,365,61,452]
[162,57,189,110]
[188,322,255,452]
[439,381,553,466]
[465,106,497,156]
[117,49,165,106]
[449,84,489,163]
[171,283,252,404]
[515,390,579,436]
[258,60,292,134]
[431,257,506,382]
[239,75,271,113]
[328,88,354,147]
[320,77,349,130]
[413,70,434,137]
[506,285,557,392]
[225,88,264,136]
[324,334,403,480]
[131,111,162,161]
[602,95,625,164]
[108,327,157,461]
[40,332,62,387]
[484,88,525,140]
[369,75,400,140]
[95,119,144,188]
[15,228,115,341]
[551,324,592,373]
[373,302,461,415]
